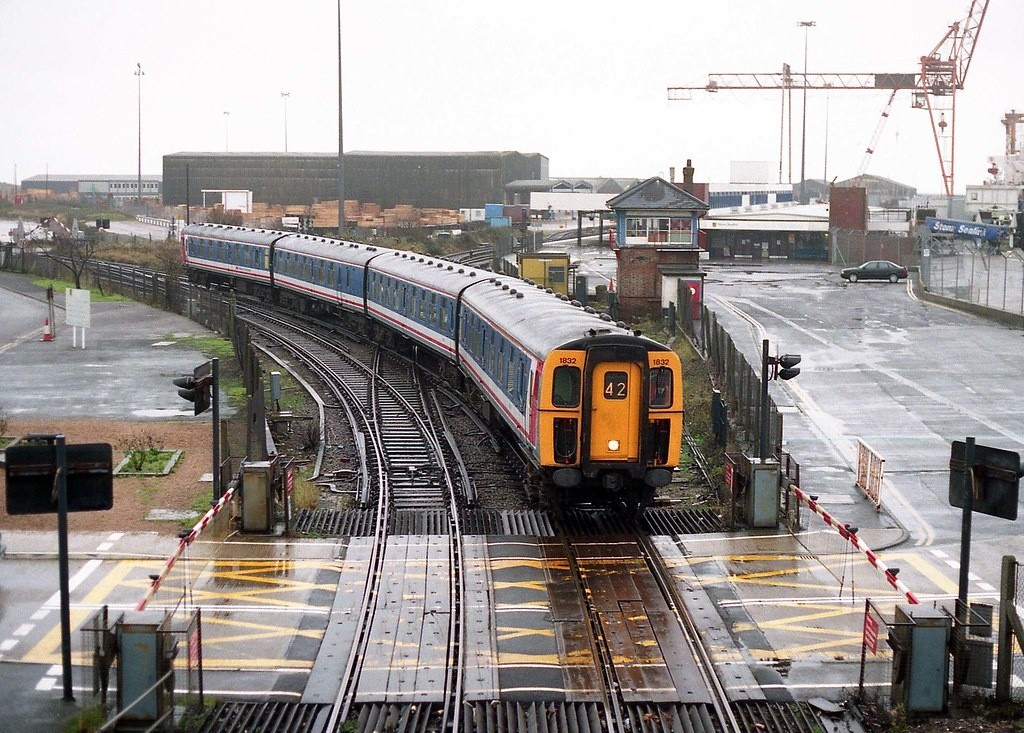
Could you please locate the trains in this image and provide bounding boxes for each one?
[178,219,686,521]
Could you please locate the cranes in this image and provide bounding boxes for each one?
[665,0,991,209]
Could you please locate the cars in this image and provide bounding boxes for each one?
[840,260,911,284]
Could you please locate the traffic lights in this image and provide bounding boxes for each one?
[174,361,212,419]
[778,352,803,383]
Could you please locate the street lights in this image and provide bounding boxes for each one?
[134,62,147,210]
[796,18,819,211]
[282,92,293,150]
[224,112,229,151]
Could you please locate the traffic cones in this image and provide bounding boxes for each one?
[39,317,54,341]
